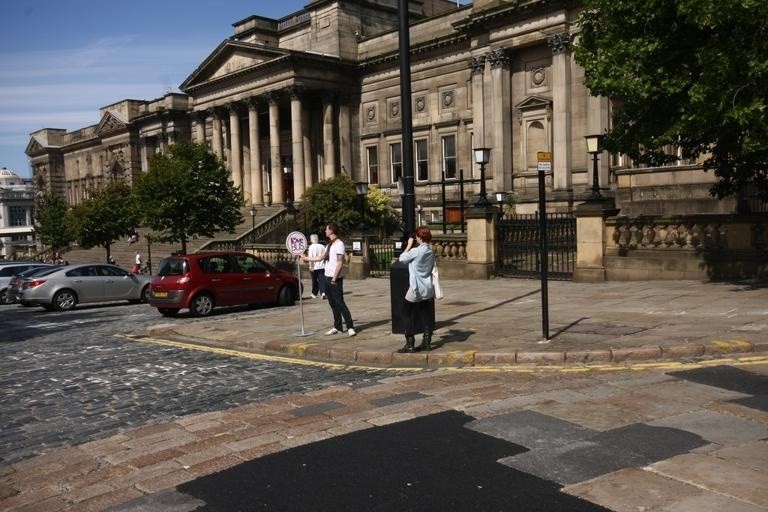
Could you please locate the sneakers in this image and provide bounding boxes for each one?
[348,328,356,336]
[309,293,316,299]
[324,327,343,335]
[320,292,326,299]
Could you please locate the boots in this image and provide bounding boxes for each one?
[398,329,416,353]
[415,327,432,351]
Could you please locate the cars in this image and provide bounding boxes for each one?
[0,258,154,311]
[151,252,303,316]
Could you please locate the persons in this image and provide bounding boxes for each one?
[131,250,150,275]
[38,250,64,265]
[398,226,436,353]
[298,221,357,337]
[308,233,328,301]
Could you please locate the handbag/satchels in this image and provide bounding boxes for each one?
[431,265,443,300]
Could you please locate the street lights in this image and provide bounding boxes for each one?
[582,134,606,200]
[472,146,492,208]
[283,165,292,206]
[249,204,257,244]
